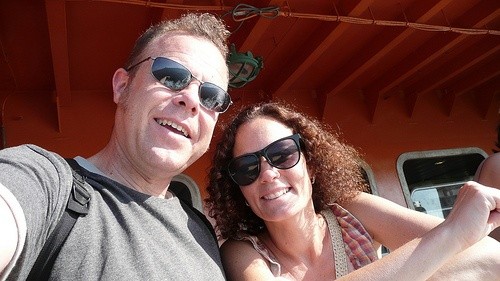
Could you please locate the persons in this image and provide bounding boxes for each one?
[0,9,233,281]
[202,100,500,281]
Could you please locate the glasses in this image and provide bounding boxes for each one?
[228,133,302,187]
[127,57,233,114]
